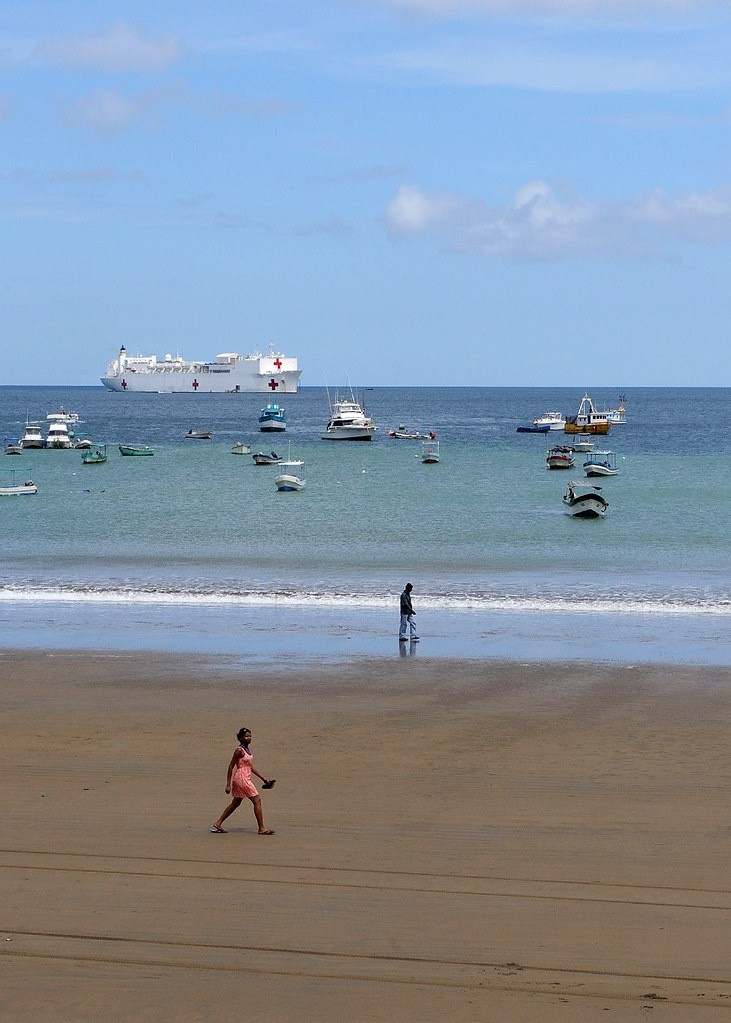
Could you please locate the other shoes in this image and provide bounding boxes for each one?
[410,636,420,639]
[399,638,408,641]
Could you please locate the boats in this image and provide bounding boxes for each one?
[273,438,306,491]
[562,480,610,517]
[573,433,595,451]
[583,449,620,476]
[183,428,213,439]
[0,479,38,496]
[253,451,282,464]
[517,425,550,433]
[421,441,440,462]
[46,405,79,425]
[388,428,437,440]
[5,408,93,456]
[231,442,251,454]
[533,410,567,430]
[320,366,380,440]
[258,396,287,431]
[546,445,575,469]
[565,389,627,434]
[120,445,156,456]
[81,450,108,463]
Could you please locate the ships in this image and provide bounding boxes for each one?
[100,343,303,393]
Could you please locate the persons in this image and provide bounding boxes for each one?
[399,583,420,642]
[212,728,278,835]
[567,488,574,501]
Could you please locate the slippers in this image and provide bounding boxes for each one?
[211,828,227,833]
[259,829,275,835]
